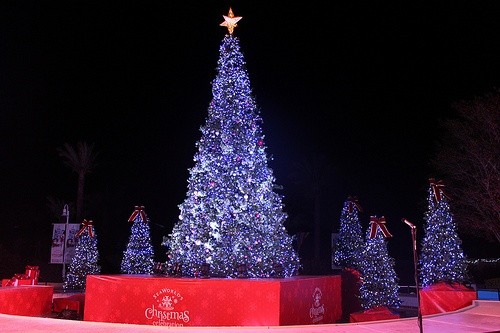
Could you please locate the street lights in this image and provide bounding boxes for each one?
[60,204,70,281]
